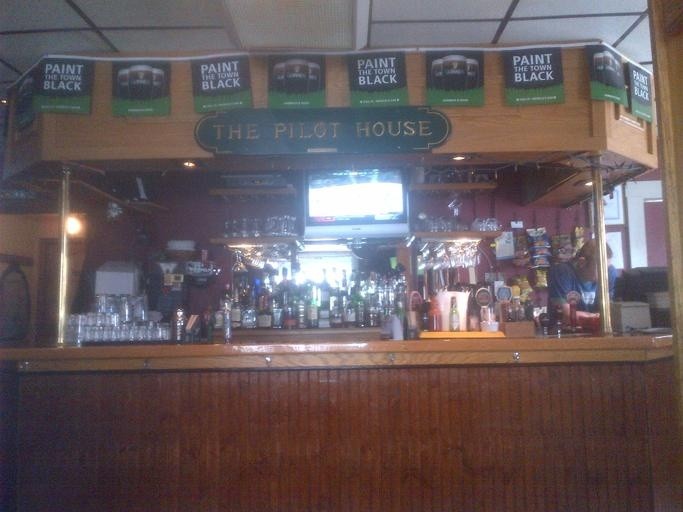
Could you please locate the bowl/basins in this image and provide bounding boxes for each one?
[479,322,499,332]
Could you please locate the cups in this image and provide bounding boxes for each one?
[470,217,498,232]
[223,215,297,238]
[64,293,170,345]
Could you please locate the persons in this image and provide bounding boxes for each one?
[547,238,617,330]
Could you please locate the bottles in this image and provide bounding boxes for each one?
[428,296,460,331]
[171,305,188,344]
[219,250,406,340]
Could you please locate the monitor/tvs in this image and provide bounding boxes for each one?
[302,169,410,239]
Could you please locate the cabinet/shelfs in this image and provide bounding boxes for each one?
[205,180,502,244]
